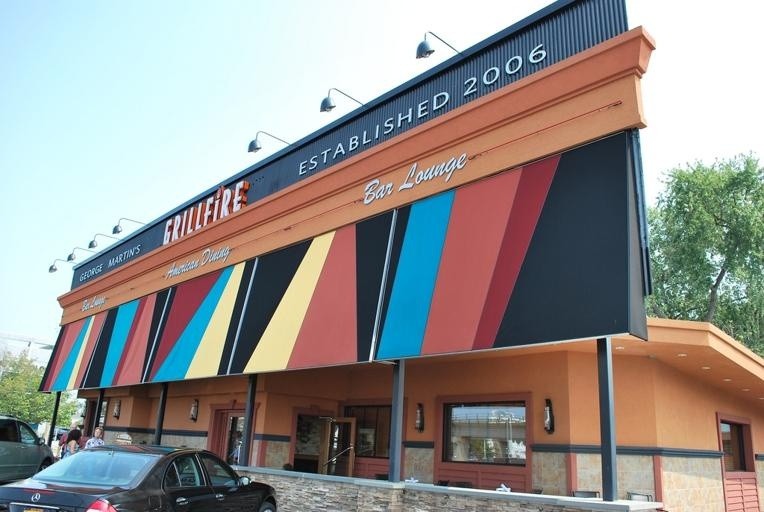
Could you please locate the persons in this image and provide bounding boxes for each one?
[59,427,105,459]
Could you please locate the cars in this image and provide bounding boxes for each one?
[0,445,277,512]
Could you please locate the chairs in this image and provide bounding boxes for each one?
[376,472,652,502]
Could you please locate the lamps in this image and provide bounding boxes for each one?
[49,259,77,273]
[189,398,198,421]
[113,399,121,419]
[416,402,424,433]
[112,219,145,235]
[416,31,462,58]
[544,397,554,435]
[320,88,364,113]
[88,234,120,248]
[66,246,97,261]
[248,132,290,153]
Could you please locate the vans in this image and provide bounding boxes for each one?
[55,428,70,441]
[0,416,54,483]
